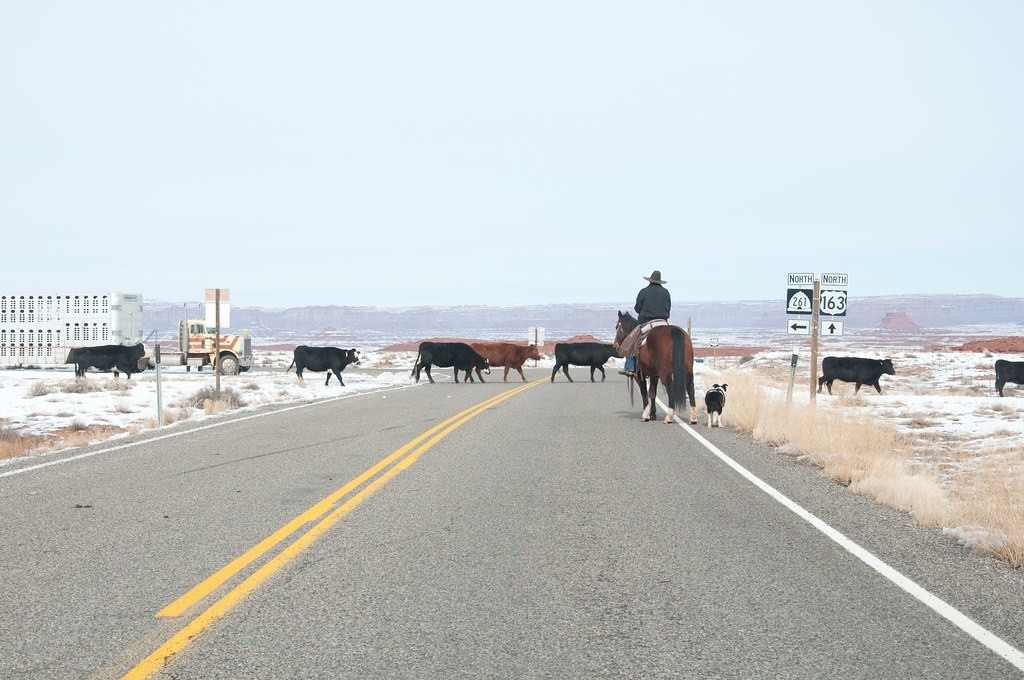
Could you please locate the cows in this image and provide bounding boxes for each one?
[551,342,624,383]
[75,342,146,381]
[994,359,1024,397]
[409,341,491,385]
[286,345,362,387]
[816,356,896,396]
[463,342,541,382]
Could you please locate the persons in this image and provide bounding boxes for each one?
[617,271,671,377]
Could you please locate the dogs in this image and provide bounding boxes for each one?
[704,383,728,429]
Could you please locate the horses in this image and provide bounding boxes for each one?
[613,309,697,424]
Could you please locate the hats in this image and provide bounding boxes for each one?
[643,271,667,284]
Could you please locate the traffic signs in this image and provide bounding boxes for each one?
[818,272,848,337]
[785,273,815,335]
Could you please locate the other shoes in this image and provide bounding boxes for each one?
[618,370,633,376]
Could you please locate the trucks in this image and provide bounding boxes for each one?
[134,302,254,376]
